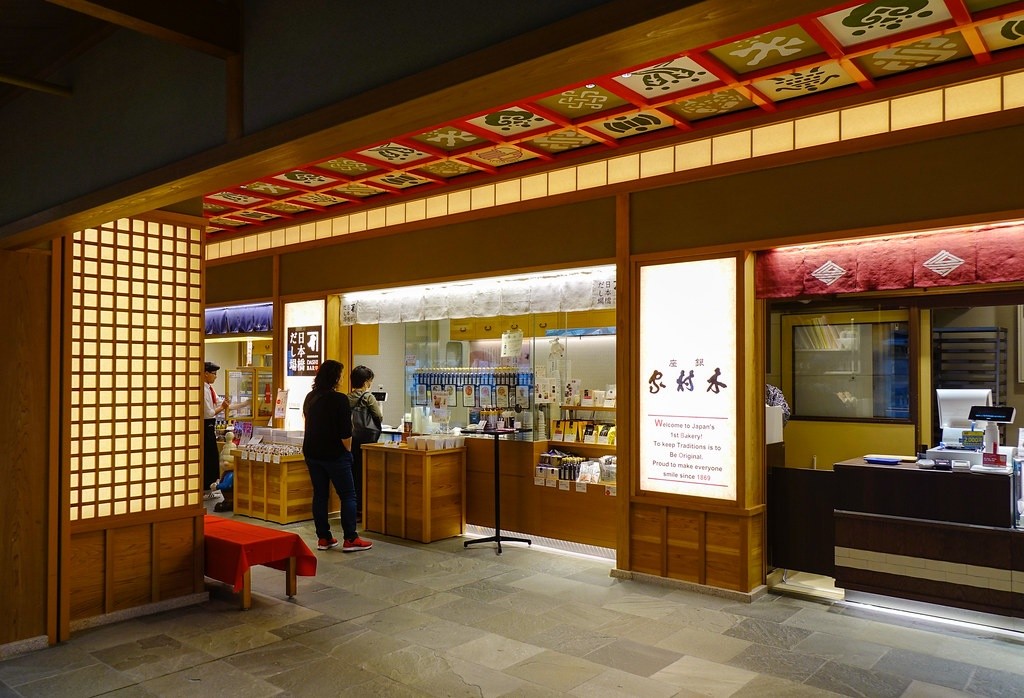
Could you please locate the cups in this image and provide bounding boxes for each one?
[594,391,606,407]
[496,421,505,430]
[521,411,546,441]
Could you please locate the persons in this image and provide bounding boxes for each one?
[210,431,237,513]
[302,360,373,551]
[203,361,251,501]
[346,365,383,523]
[765,383,791,429]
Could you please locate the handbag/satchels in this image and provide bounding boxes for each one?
[351,392,381,443]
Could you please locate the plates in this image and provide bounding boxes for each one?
[864,458,903,465]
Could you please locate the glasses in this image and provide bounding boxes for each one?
[208,371,216,375]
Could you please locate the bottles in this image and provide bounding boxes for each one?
[538,453,586,482]
[215,420,235,435]
[480,404,510,430]
[413,367,532,387]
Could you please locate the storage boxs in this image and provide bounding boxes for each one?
[253,427,304,445]
[407,436,465,450]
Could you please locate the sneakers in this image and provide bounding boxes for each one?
[317,539,338,550]
[342,538,373,550]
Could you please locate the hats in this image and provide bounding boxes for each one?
[204,361,220,372]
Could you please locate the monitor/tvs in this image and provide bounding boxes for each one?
[936,388,992,448]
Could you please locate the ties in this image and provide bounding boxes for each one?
[209,385,217,403]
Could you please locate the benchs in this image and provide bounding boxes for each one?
[203,515,298,611]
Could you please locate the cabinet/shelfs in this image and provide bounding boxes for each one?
[225,367,273,428]
[888,326,1008,446]
[450,309,617,340]
[535,406,616,486]
